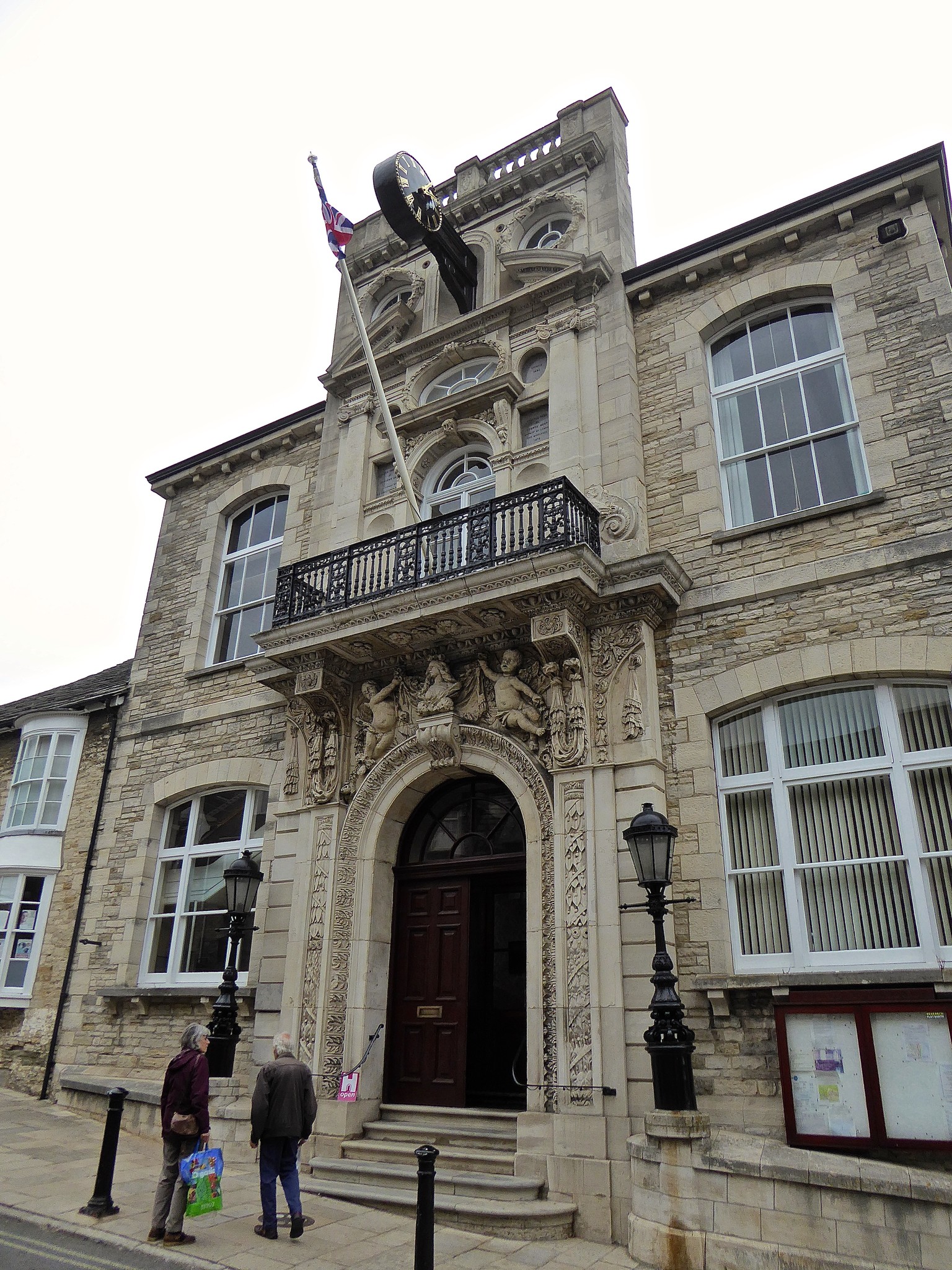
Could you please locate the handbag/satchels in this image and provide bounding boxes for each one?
[170,1112,198,1135]
[181,1148,224,1218]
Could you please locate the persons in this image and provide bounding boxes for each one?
[477,647,546,752]
[249,1032,318,1241]
[400,660,461,717]
[145,1021,212,1247]
[359,670,403,774]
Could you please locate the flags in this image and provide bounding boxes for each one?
[309,155,355,273]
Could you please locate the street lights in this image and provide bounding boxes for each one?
[621,801,698,1111]
[202,849,265,1078]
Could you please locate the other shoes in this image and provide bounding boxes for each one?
[147,1227,166,1242]
[255,1225,279,1240]
[290,1211,304,1238]
[163,1231,195,1246]
